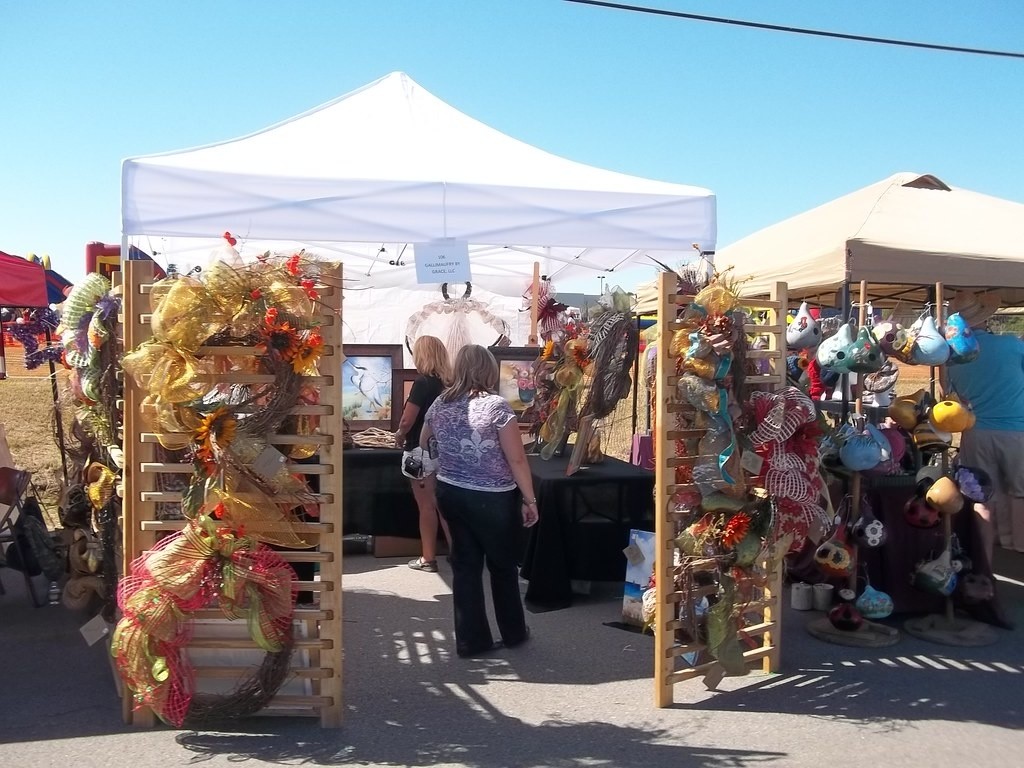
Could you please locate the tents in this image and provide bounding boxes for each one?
[0,251,69,488]
[120,72,716,664]
[631,167,1024,469]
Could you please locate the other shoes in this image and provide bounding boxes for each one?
[296,597,322,610]
[447,556,452,562]
[408,554,438,571]
[518,626,530,645]
[458,638,502,656]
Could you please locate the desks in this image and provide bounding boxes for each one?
[782,462,999,615]
[633,433,653,467]
[814,399,891,420]
[343,446,447,542]
[523,442,655,614]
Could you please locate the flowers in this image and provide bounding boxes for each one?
[261,307,326,373]
[541,340,555,360]
[573,347,590,368]
[565,320,586,339]
[196,405,241,448]
[719,511,751,548]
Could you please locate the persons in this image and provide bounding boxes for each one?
[941,290,1024,570]
[420,345,538,657]
[395,335,453,573]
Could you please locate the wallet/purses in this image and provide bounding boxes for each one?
[427,434,439,460]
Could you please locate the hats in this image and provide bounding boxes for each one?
[947,289,1002,327]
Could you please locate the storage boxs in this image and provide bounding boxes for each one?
[370,536,443,556]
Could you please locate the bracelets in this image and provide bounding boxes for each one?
[523,498,537,506]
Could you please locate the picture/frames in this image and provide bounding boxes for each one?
[342,343,404,431]
[488,345,545,426]
[390,368,419,432]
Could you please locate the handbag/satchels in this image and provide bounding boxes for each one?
[404,457,423,478]
[5,472,66,580]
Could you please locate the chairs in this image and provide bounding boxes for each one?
[0,465,46,612]
